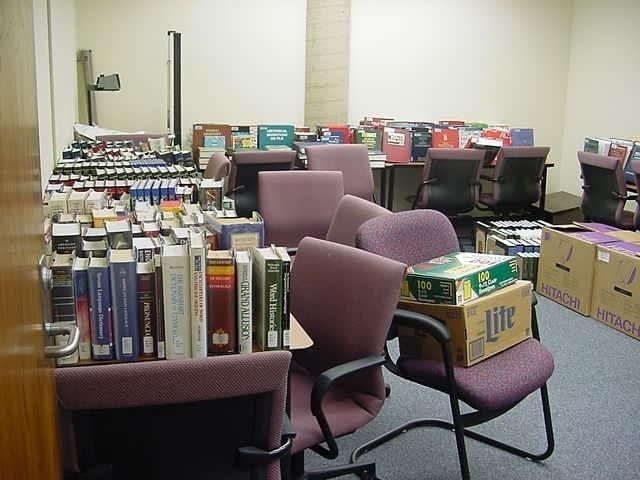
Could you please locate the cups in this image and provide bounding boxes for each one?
[400,280,410,298]
[463,278,472,301]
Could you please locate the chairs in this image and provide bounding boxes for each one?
[52,349,293,480]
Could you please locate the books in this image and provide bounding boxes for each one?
[42,115,553,366]
[583,136,640,184]
